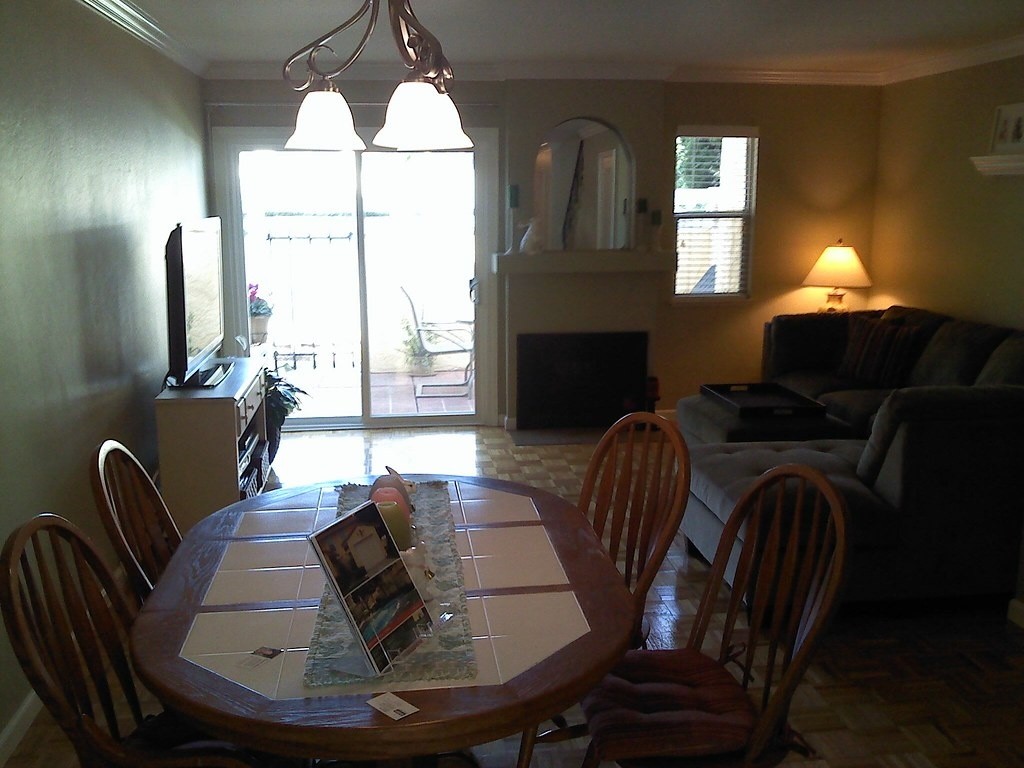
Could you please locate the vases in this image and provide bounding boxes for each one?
[251,315,271,344]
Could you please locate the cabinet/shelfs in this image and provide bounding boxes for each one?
[154,357,271,536]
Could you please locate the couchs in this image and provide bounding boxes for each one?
[673,305,1024,627]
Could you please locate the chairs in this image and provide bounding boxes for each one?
[1,513,269,768]
[399,284,476,400]
[89,438,183,609]
[576,412,690,651]
[517,463,850,768]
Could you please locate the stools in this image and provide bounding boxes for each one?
[676,395,854,444]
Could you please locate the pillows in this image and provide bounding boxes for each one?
[836,314,923,389]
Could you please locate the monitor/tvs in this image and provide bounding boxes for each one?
[164,216,235,388]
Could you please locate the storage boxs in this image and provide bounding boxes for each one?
[240,468,258,499]
[250,441,269,487]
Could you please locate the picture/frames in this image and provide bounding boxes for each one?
[989,103,1024,155]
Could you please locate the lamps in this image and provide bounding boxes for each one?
[802,238,872,314]
[281,0,476,149]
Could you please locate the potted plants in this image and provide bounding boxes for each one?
[263,363,309,465]
[401,316,442,377]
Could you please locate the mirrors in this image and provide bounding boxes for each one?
[532,117,631,251]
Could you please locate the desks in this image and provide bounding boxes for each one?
[128,475,638,768]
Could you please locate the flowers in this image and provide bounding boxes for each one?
[249,283,273,317]
